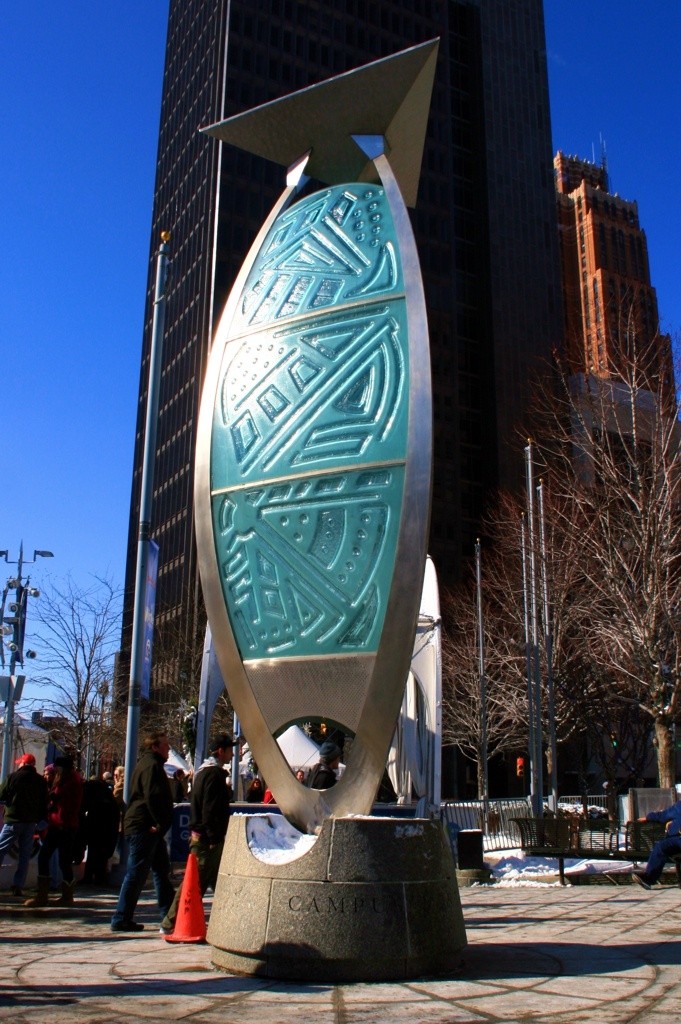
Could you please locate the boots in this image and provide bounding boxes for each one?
[23,876,51,906]
[50,877,76,906]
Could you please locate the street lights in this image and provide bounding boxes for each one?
[0,538,54,789]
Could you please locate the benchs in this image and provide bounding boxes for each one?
[508,817,681,886]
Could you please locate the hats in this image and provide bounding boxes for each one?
[15,754,36,764]
[53,756,73,766]
[208,734,240,749]
[44,764,55,771]
[320,742,340,759]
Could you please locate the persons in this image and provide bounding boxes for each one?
[631,784,681,889]
[161,731,240,933]
[111,733,175,932]
[0,741,398,912]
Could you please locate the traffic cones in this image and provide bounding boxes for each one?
[163,854,207,944]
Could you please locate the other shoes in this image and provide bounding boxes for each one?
[12,886,23,896]
[111,920,144,931]
[632,872,653,890]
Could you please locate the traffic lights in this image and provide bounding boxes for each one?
[610,732,618,749]
[517,757,524,777]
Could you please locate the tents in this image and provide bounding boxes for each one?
[260,724,345,788]
